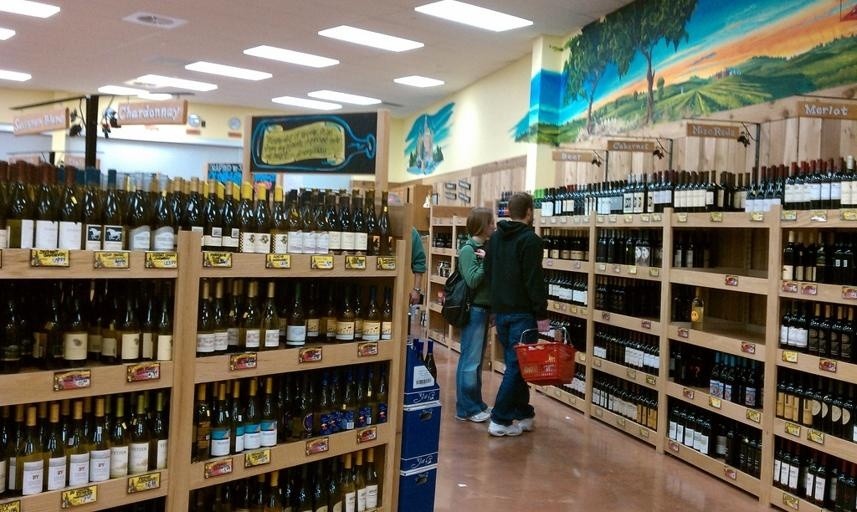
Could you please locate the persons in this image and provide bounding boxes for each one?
[409,225,428,338]
[483,193,551,437]
[454,206,495,424]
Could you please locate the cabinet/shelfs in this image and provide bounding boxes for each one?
[0,203,412,511]
[427,205,856,512]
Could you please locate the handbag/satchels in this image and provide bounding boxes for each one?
[442,270,473,327]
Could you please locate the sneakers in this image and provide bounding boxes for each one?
[457,407,534,437]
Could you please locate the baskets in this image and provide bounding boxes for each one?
[514,327,575,385]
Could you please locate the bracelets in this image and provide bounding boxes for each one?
[412,286,422,293]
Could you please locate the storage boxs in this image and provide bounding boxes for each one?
[397,341,443,512]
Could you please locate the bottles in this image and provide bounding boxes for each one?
[191,361,388,461]
[0,392,168,499]
[0,158,396,253]
[437,260,451,276]
[546,270,691,320]
[411,338,436,383]
[691,287,704,330]
[431,232,465,248]
[495,155,857,219]
[541,229,711,268]
[768,230,856,512]
[536,315,763,483]
[195,277,395,356]
[1,279,175,374]
[188,447,378,512]
